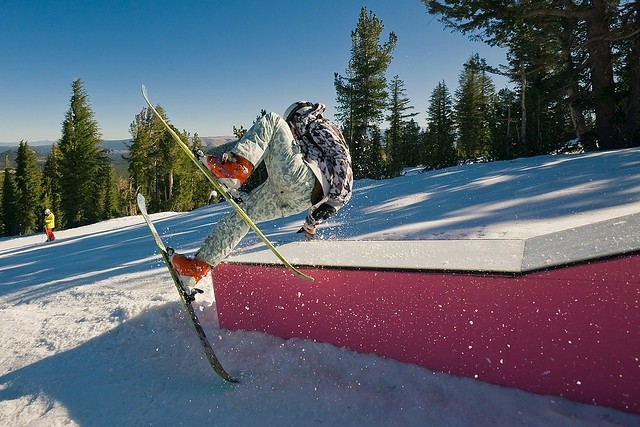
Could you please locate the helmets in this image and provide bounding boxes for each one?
[283,100,313,123]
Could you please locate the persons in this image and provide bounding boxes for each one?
[166,100,353,292]
[207,186,217,205]
[42,208,56,241]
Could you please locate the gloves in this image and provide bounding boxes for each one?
[296,220,316,240]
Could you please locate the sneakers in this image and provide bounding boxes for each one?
[168,252,212,295]
[201,153,255,193]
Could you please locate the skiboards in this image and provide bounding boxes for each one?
[136,83,315,382]
